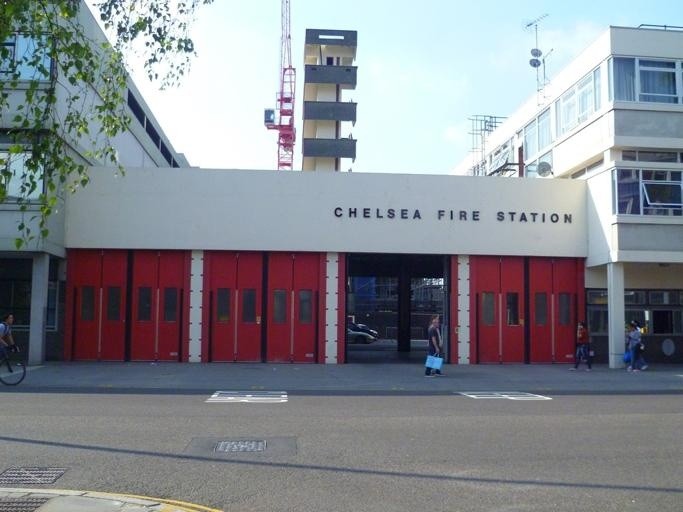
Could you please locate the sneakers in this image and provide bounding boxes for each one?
[626,364,649,373]
[424,372,446,377]
[568,367,593,372]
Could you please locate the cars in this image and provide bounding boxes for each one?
[348,324,380,344]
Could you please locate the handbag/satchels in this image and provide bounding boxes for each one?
[423,352,444,370]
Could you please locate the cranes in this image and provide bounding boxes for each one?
[261,0,296,170]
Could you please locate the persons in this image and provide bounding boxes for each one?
[424,313,446,379]
[625,325,641,373]
[629,319,648,370]
[0,314,17,359]
[566,320,594,371]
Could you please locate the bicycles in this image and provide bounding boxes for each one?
[0,344,26,385]
